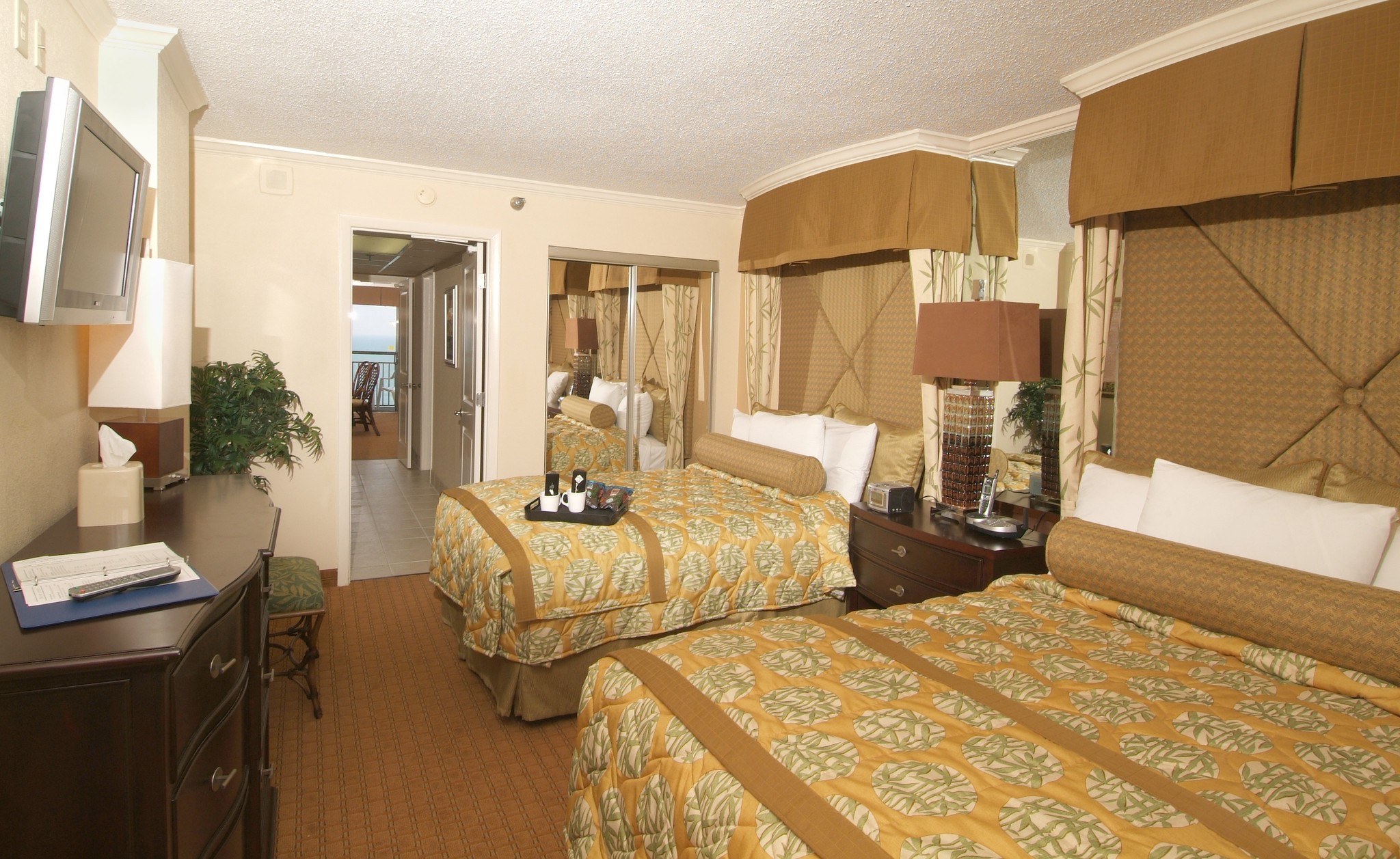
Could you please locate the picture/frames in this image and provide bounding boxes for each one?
[444,285,458,368]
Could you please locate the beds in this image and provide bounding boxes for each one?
[546,395,666,472]
[567,518,1400,859]
[428,432,857,720]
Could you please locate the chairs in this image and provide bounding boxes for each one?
[351,361,396,437]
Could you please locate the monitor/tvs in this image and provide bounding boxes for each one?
[0,76,152,325]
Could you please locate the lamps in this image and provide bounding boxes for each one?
[564,318,599,400]
[1040,308,1067,497]
[912,300,1040,524]
[510,197,526,211]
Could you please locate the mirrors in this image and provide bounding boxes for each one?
[967,133,1125,510]
[544,244,719,478]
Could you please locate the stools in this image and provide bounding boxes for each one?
[267,556,326,719]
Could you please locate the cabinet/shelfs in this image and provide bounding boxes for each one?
[0,473,279,859]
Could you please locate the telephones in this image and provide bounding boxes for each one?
[966,470,1028,539]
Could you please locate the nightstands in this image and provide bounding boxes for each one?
[845,500,1049,615]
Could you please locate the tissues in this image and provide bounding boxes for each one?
[75,421,145,527]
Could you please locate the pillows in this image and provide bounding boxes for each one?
[1045,450,1400,683]
[694,404,925,503]
[547,360,671,446]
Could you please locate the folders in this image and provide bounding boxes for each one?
[0,539,219,629]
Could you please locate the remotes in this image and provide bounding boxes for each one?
[69,565,181,599]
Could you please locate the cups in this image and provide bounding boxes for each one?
[540,491,561,512]
[561,489,588,513]
